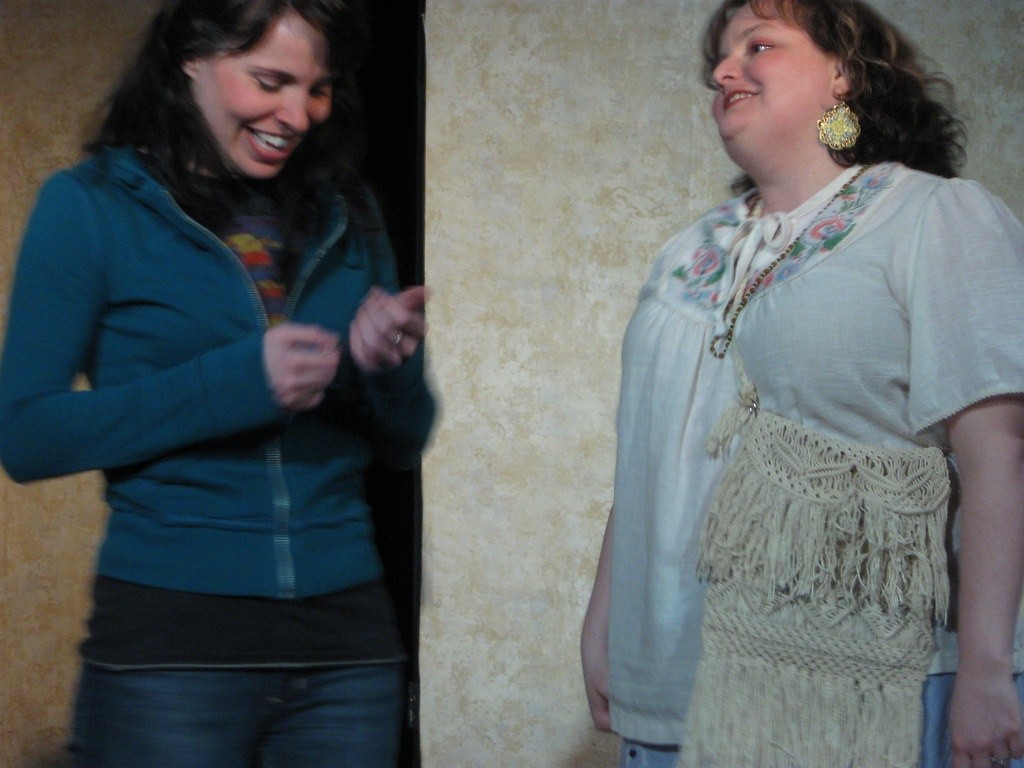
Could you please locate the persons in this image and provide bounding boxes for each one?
[1,0,437,768]
[581,0,1023,768]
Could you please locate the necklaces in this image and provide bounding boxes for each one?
[710,164,869,358]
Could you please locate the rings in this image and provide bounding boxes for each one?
[395,333,403,346]
